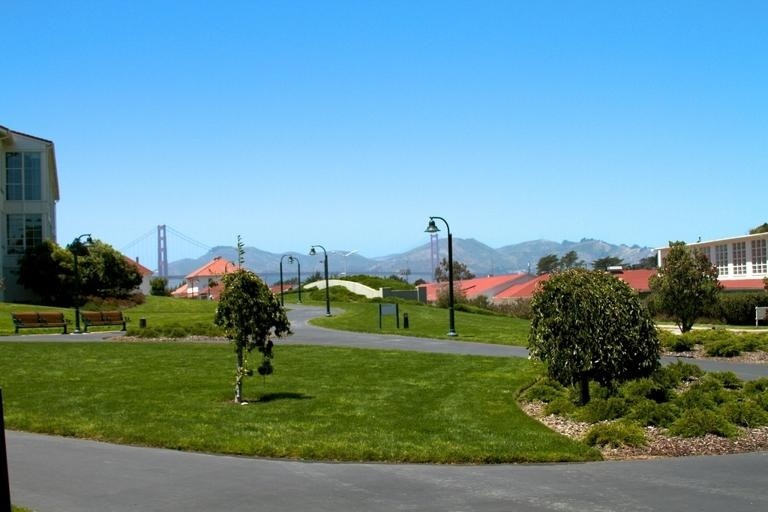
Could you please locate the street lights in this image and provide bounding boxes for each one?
[68,230,93,334]
[278,244,335,317]
[423,215,457,337]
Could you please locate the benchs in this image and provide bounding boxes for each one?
[10,312,68,334]
[80,311,126,332]
